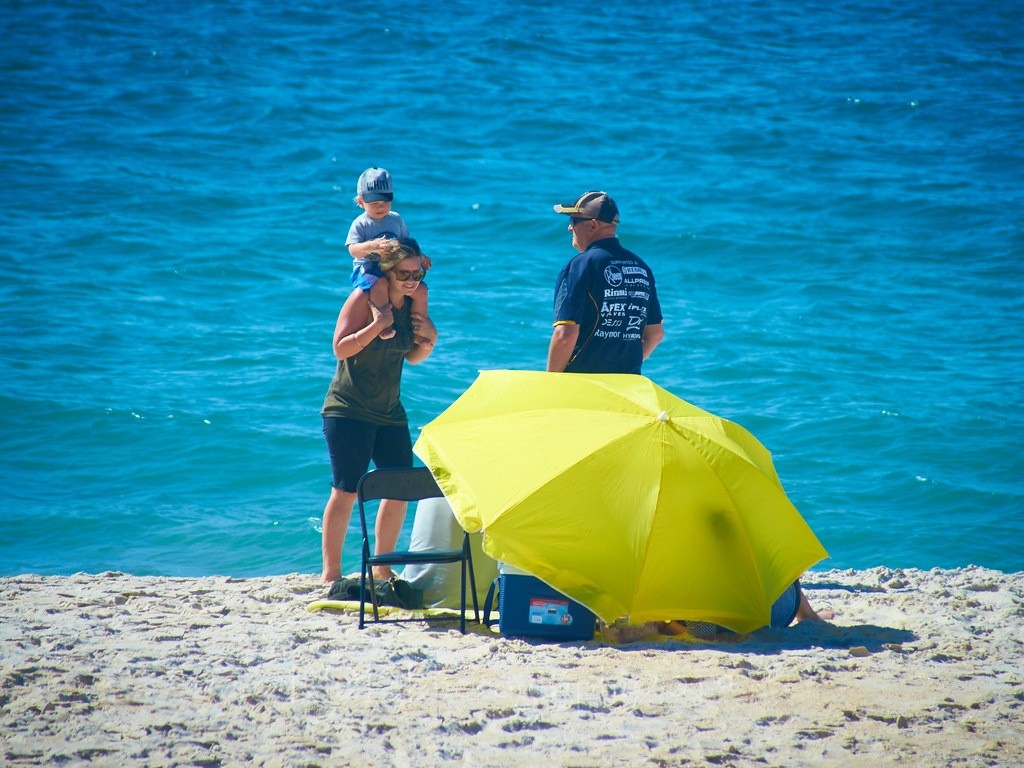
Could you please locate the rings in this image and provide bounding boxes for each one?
[414,326,416,329]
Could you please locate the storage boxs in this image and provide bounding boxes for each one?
[499,559,597,640]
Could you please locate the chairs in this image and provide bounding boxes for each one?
[357,465,480,635]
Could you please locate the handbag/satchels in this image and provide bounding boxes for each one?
[328,577,423,609]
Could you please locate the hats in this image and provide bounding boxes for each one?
[554,190,621,216]
[357,167,393,204]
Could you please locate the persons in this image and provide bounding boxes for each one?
[546,190,664,374]
[320,237,437,588]
[346,166,433,348]
[659,577,834,637]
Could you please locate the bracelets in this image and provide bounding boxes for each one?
[354,334,365,348]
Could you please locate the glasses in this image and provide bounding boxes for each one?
[390,267,425,281]
[569,215,598,228]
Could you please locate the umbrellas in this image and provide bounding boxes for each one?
[412,367,829,636]
